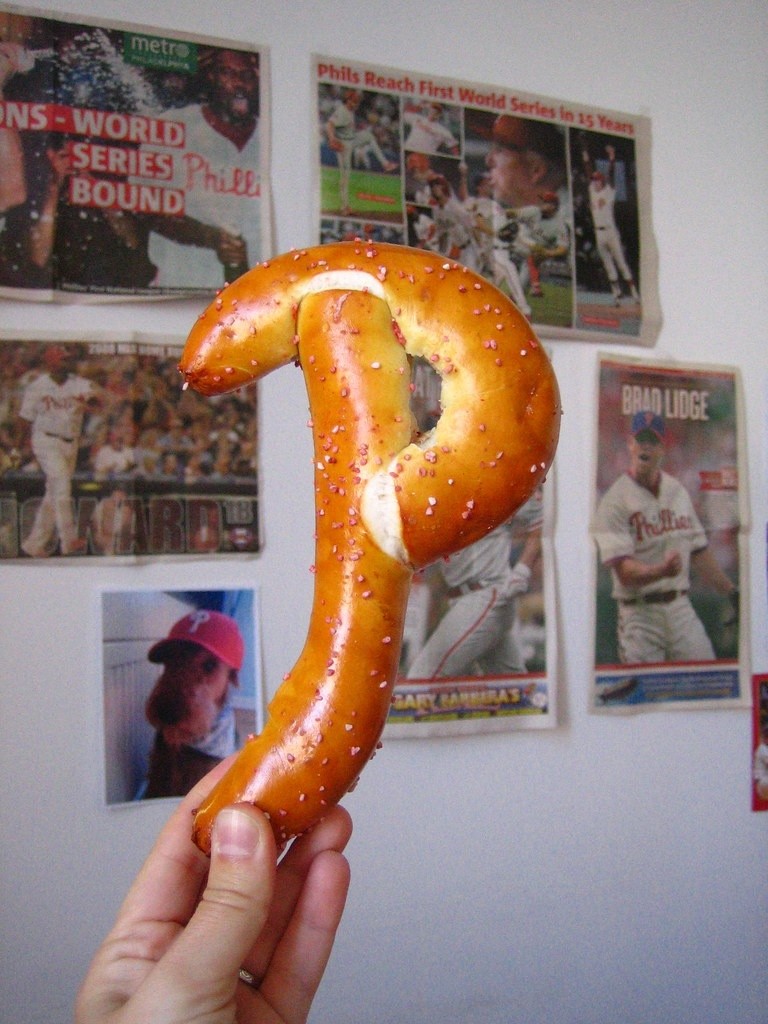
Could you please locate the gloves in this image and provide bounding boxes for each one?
[498,565,533,598]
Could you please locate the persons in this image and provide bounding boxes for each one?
[0,13,262,282]
[0,341,256,554]
[319,84,638,324]
[397,373,542,680]
[593,411,736,664]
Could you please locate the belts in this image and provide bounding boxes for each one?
[622,589,690,605]
[45,432,74,443]
[445,582,484,597]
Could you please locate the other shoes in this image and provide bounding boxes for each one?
[384,163,399,172]
[338,207,360,216]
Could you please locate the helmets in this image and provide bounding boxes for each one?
[427,174,450,197]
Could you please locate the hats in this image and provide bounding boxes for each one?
[590,171,605,181]
[147,611,244,673]
[472,114,559,154]
[45,344,73,361]
[538,191,561,202]
[632,411,666,443]
[432,104,442,112]
[344,91,361,103]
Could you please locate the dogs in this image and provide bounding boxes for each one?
[141,610,245,800]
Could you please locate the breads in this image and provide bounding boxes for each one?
[179,240,561,858]
